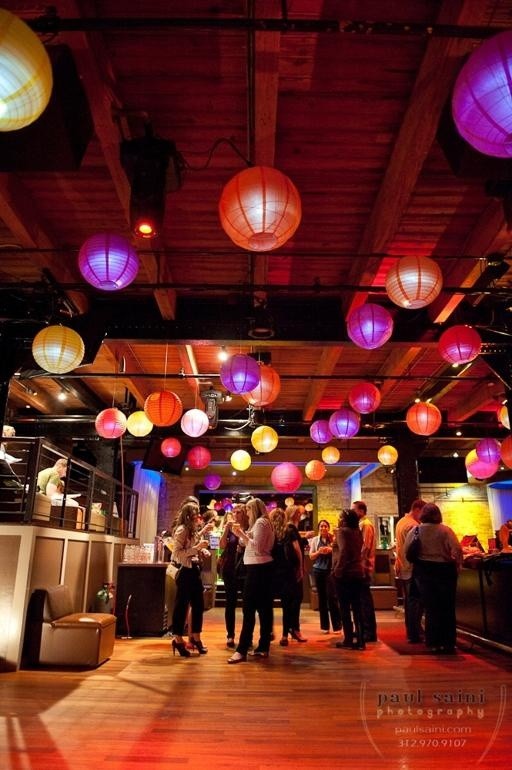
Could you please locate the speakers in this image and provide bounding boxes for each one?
[417,457,468,483]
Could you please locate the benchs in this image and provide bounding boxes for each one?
[309,585,398,611]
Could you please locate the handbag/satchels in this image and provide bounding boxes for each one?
[406,539,420,563]
[166,564,180,581]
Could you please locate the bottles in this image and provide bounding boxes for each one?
[383,538,387,549]
[124,544,155,564]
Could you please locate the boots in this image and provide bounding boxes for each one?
[336,622,353,649]
[353,622,365,650]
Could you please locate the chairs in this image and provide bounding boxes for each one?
[39,584,117,670]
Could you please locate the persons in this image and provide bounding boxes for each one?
[0,424,464,663]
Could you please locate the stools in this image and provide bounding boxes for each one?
[203,585,217,610]
[1,490,131,538]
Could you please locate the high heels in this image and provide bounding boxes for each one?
[190,636,207,654]
[172,639,190,656]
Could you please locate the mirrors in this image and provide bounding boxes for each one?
[374,512,400,551]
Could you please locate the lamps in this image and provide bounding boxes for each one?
[0,1,512,494]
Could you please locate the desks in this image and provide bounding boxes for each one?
[118,562,179,637]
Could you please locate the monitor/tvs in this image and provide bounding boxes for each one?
[141,437,191,475]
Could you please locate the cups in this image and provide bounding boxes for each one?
[233,524,240,534]
[487,538,496,551]
[208,523,213,532]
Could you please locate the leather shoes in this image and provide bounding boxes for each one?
[227,630,307,664]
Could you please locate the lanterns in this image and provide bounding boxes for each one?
[1,10,512,496]
